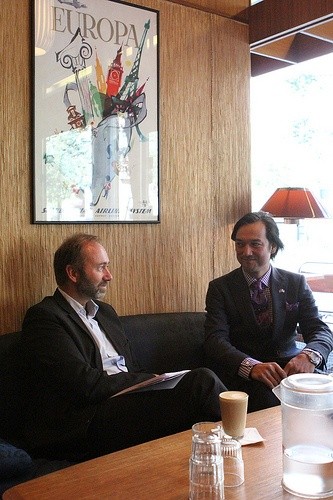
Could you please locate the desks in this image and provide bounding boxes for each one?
[3,405,333,500]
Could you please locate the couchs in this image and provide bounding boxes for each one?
[0,312,210,495]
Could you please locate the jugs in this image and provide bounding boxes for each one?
[271,372,333,499]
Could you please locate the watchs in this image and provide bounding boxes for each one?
[300,349,320,367]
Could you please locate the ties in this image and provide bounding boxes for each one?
[253,280,270,324]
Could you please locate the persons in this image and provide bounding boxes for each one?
[21,233,228,465]
[204,212,333,414]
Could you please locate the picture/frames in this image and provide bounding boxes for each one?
[30,0,161,224]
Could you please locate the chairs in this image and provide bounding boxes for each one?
[298,261,333,324]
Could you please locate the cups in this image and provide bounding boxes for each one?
[219,390,249,440]
[188,421,225,500]
[220,439,244,488]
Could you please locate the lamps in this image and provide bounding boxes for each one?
[259,186,328,227]
